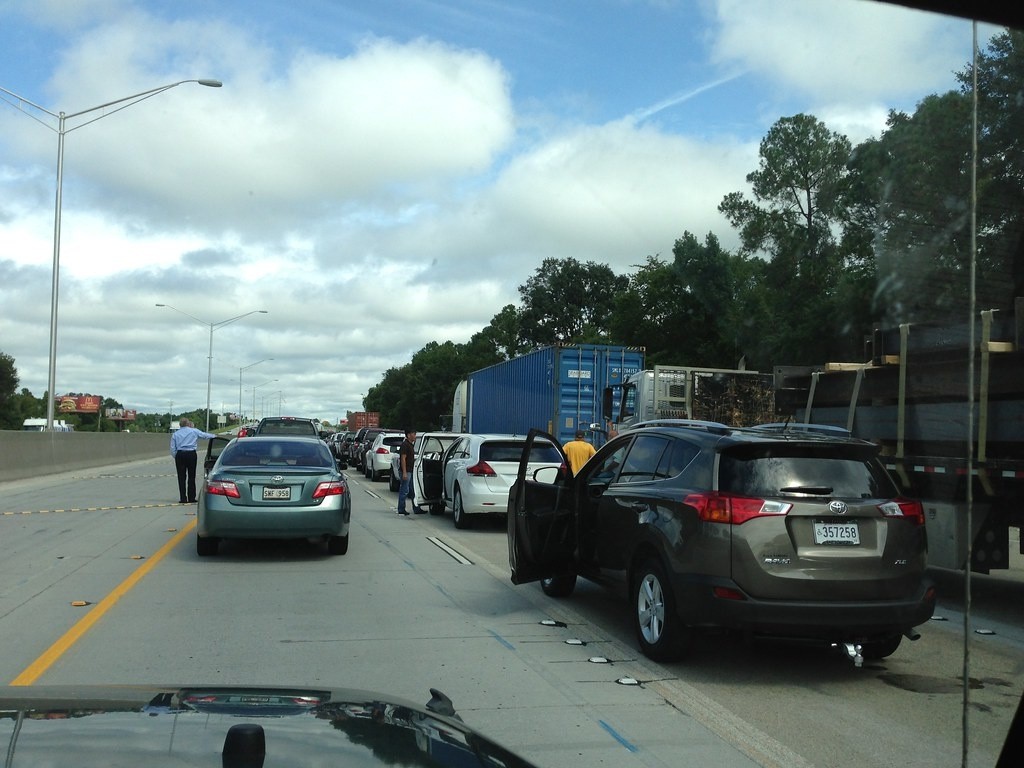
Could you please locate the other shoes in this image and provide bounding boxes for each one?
[398,510,409,515]
[188,499,198,503]
[415,509,428,515]
[179,500,188,503]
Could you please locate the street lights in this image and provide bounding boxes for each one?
[239,357,275,433]
[155,303,269,433]
[1,77,225,432]
[252,379,279,422]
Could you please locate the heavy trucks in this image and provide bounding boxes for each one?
[451,341,647,453]
[23,417,74,433]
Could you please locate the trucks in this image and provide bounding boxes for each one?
[601,365,778,435]
[347,412,380,432]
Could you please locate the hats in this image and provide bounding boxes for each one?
[575,430,585,438]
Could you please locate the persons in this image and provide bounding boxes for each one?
[561,429,597,478]
[169,417,219,504]
[398,427,428,515]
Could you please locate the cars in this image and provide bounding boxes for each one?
[197,436,351,556]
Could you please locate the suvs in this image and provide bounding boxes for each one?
[504,417,935,663]
[413,434,567,530]
[322,426,471,500]
[246,416,329,442]
